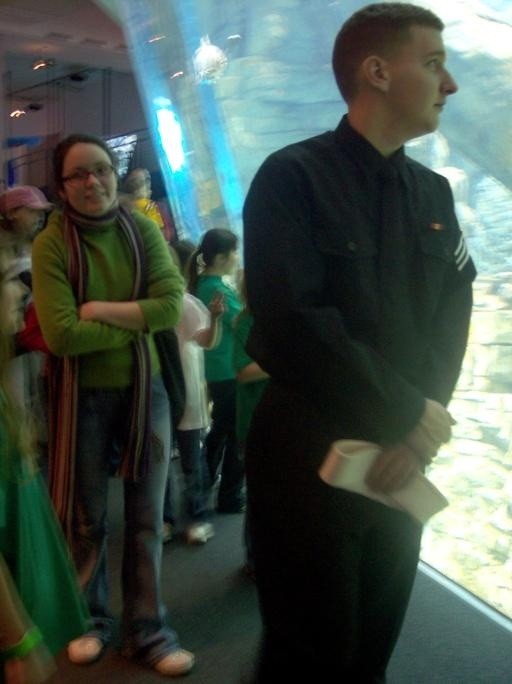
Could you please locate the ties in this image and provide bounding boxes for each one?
[372,162,428,379]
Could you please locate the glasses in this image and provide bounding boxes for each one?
[60,162,118,184]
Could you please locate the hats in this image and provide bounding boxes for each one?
[0,183,56,215]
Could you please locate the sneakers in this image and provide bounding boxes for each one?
[154,441,251,547]
[66,610,114,666]
[120,627,200,680]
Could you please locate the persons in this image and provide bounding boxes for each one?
[3,171,264,541]
[239,3,480,684]
[33,137,195,675]
[2,235,91,684]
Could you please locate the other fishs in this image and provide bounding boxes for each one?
[191,35,229,84]
